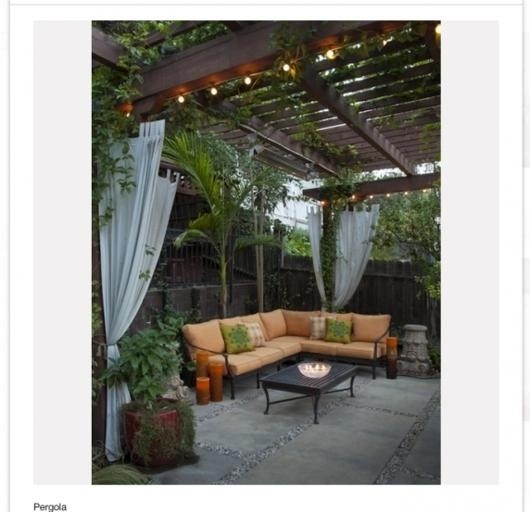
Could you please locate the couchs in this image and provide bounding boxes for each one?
[181,308,393,399]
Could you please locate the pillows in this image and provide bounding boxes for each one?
[183,308,392,360]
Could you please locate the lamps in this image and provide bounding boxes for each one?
[252,140,266,156]
[114,101,133,118]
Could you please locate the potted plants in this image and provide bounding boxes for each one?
[96,303,200,474]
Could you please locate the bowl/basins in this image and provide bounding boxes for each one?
[297,362,331,379]
